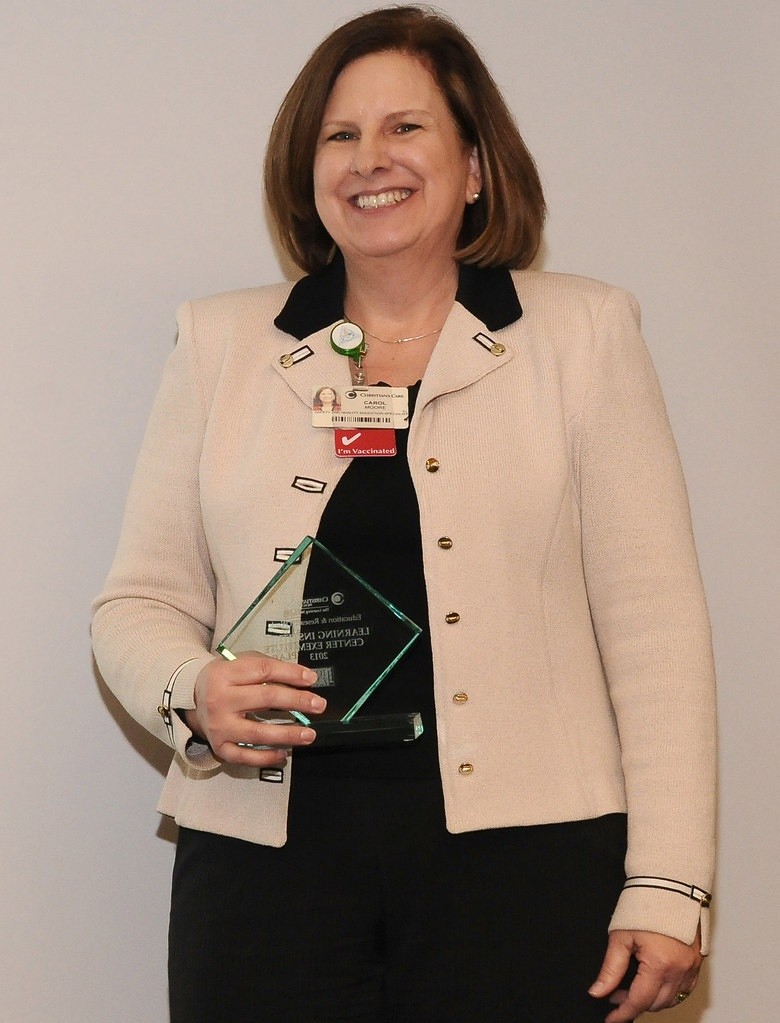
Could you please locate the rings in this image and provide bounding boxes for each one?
[674,992,689,1003]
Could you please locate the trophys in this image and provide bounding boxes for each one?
[214,537,423,750]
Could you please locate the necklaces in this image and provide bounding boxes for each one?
[344,313,442,343]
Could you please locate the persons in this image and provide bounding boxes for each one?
[89,8,718,1023]
[312,387,341,413]
